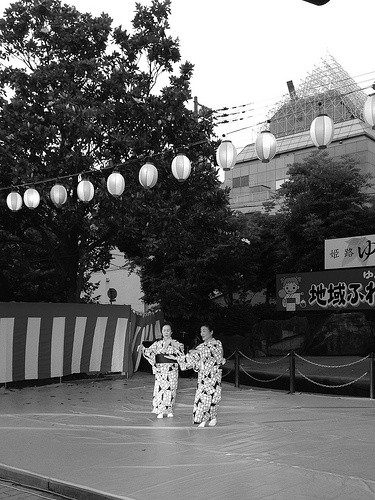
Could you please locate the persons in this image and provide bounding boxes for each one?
[137,322,184,418]
[165,319,226,427]
